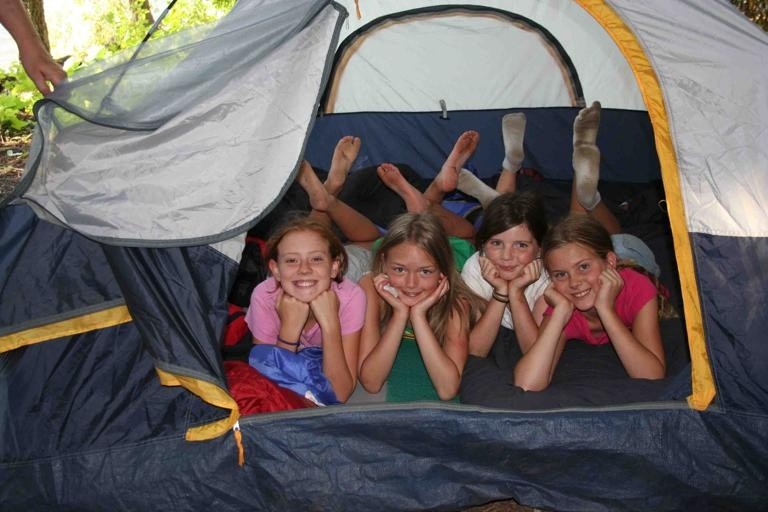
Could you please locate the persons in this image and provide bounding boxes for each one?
[355,127,481,401]
[0,4,69,101]
[243,129,388,407]
[453,107,561,363]
[511,98,667,393]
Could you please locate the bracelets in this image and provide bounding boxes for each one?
[493,291,510,298]
[491,293,510,304]
[275,334,300,349]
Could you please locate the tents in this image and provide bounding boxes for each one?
[0,1,767,511]
